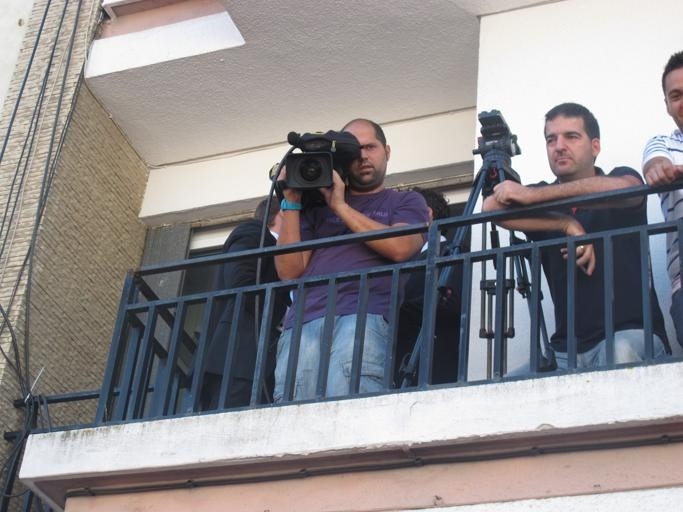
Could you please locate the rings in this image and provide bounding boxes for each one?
[579,245,585,251]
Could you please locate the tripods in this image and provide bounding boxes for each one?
[400,154,556,388]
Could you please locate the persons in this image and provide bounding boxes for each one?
[480,103,672,379]
[182,194,292,414]
[272,118,428,406]
[640,50,682,354]
[392,184,465,388]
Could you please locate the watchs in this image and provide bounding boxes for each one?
[277,198,304,214]
